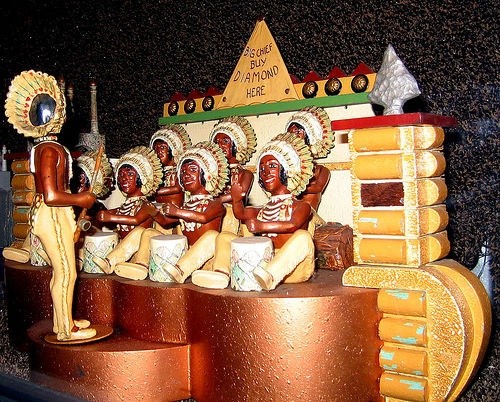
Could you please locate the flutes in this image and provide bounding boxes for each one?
[72,145,104,244]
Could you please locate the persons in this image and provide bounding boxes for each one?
[76,146,164,274]
[285,104,337,241]
[146,122,193,208]
[114,140,228,284]
[208,116,257,235]
[7,70,97,340]
[191,140,316,292]
[4,147,115,263]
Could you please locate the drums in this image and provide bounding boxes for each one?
[150,234,188,282]
[83,232,118,274]
[230,236,274,292]
